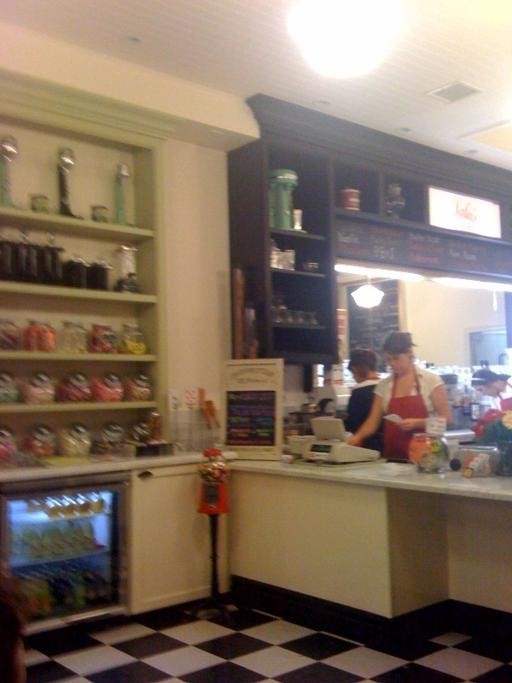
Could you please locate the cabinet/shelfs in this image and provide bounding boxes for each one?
[0,203,162,414]
[225,134,509,362]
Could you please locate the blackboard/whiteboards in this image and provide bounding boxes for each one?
[345,279,405,373]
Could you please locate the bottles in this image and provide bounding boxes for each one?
[387,182,406,218]
[0,318,158,466]
[409,434,450,472]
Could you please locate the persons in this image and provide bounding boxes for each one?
[346,332,452,457]
[471,370,512,411]
[347,350,384,451]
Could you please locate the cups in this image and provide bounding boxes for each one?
[339,187,362,212]
[291,209,304,229]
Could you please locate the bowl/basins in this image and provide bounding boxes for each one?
[454,444,502,478]
[300,260,321,272]
